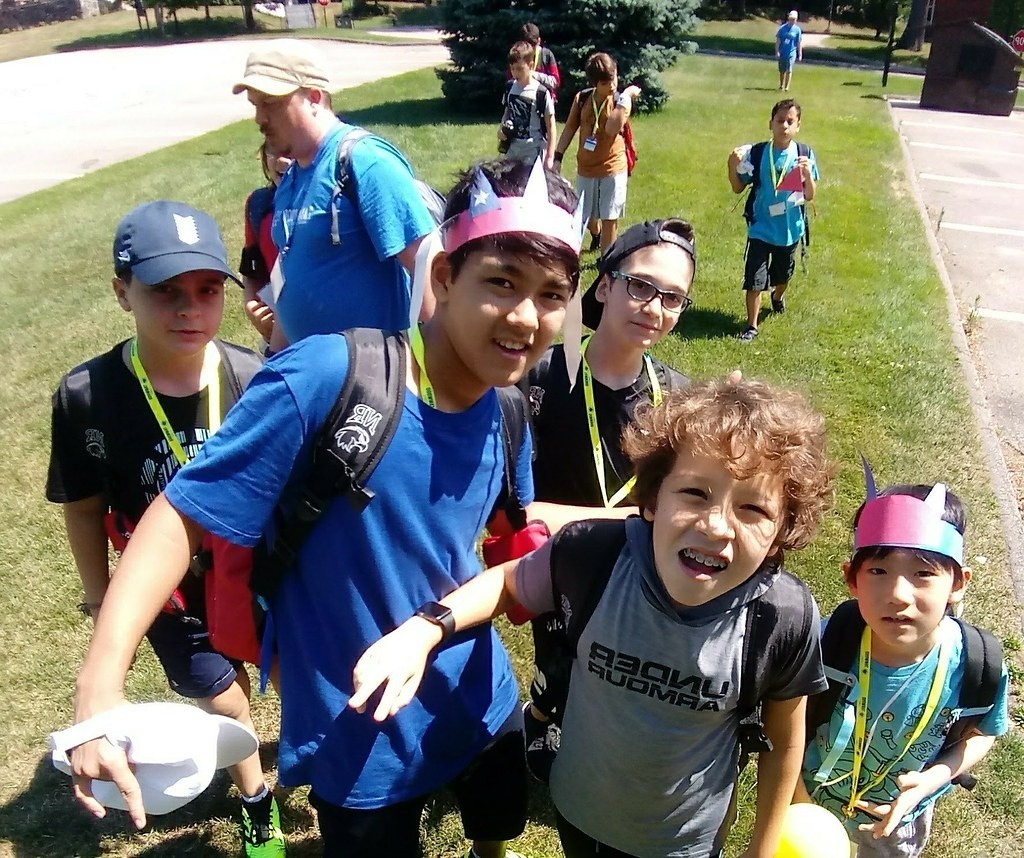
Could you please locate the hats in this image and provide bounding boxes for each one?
[113,201,246,290]
[581,218,696,331]
[48,703,259,816]
[788,11,798,19]
[232,52,332,96]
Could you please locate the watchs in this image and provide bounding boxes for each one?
[412,601,455,641]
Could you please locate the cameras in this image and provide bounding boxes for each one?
[498,126,513,154]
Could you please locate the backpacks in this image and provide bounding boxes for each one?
[195,329,529,666]
[577,88,639,177]
[335,129,447,232]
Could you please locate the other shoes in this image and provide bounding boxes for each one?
[779,87,783,89]
[462,847,527,858]
[740,325,759,342]
[771,289,785,314]
[589,230,602,252]
[785,88,787,91]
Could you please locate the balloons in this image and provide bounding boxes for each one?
[770,803,851,858]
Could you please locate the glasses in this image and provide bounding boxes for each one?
[612,270,693,313]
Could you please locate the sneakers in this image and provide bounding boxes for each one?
[241,788,286,858]
[522,701,561,784]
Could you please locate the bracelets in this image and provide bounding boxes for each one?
[88,601,104,613]
[264,347,277,358]
[617,93,630,110]
[554,151,565,162]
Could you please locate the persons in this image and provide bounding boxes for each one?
[348,377,830,858]
[486,215,742,784]
[232,40,445,363]
[71,155,641,858]
[728,100,819,341]
[506,24,560,121]
[778,486,1011,858]
[551,53,642,266]
[240,137,305,355]
[497,40,557,172]
[46,199,310,858]
[776,10,802,91]
